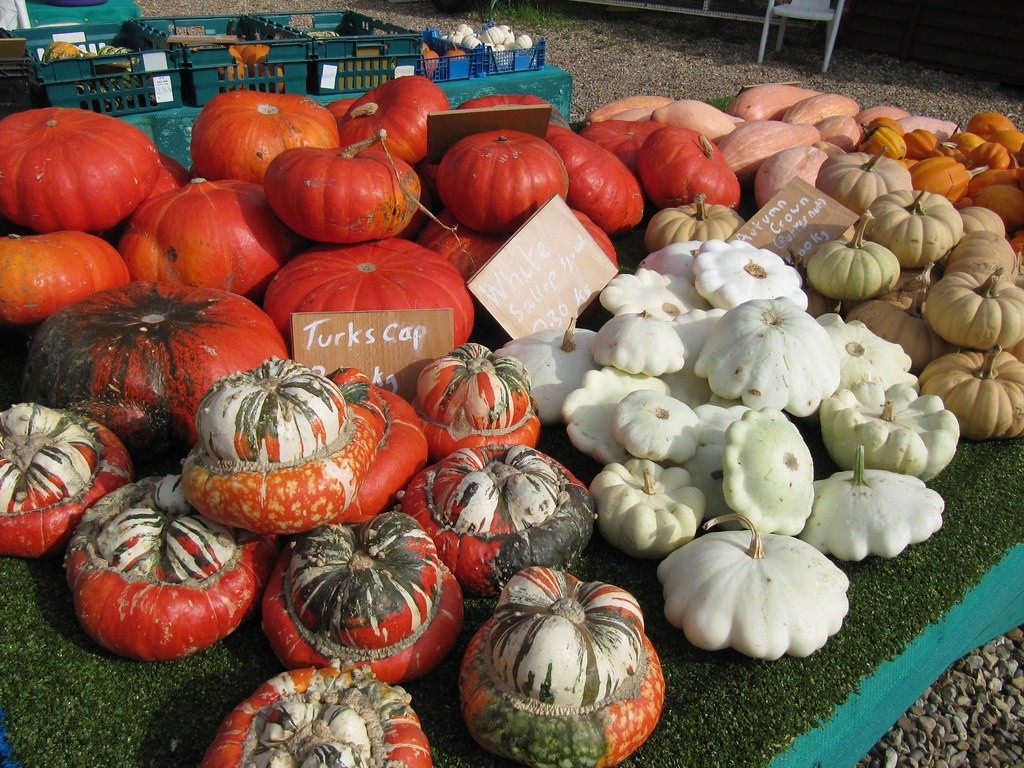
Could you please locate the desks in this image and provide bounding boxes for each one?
[124,65,572,172]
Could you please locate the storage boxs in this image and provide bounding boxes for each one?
[130,12,314,107]
[249,9,424,96]
[12,19,183,116]
[418,28,483,83]
[429,21,546,78]
[0,26,35,119]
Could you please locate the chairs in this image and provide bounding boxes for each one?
[756,0,845,71]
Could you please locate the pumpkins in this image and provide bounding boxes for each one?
[0,22,1024,768]
